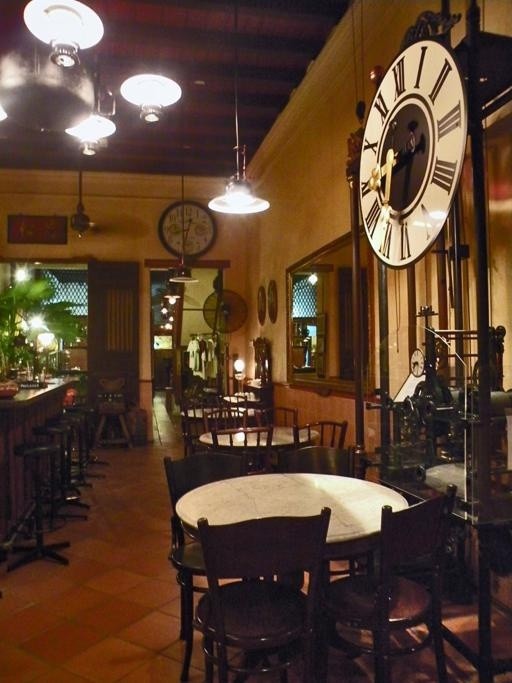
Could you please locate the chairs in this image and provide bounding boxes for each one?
[95,377,134,450]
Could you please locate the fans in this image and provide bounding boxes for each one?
[26,166,151,244]
[203,289,248,396]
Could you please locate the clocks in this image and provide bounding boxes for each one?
[410,349,425,377]
[357,40,468,270]
[158,200,217,260]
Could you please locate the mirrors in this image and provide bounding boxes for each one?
[285,224,375,397]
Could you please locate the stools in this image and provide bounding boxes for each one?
[1,405,111,572]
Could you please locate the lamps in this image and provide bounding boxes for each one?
[23,0,105,70]
[119,74,182,129]
[308,272,319,287]
[64,52,117,156]
[158,294,183,330]
[168,1,198,283]
[233,359,246,392]
[208,0,271,214]
[0,105,8,122]
